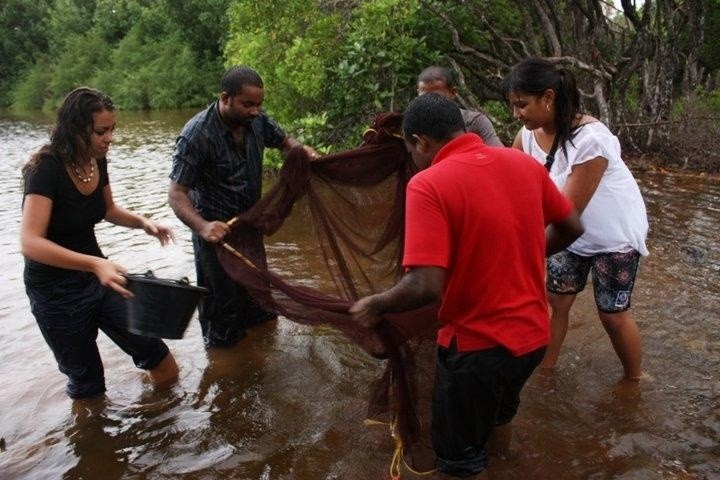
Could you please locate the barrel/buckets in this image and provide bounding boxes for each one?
[120,269,210,340]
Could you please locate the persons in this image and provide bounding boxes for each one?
[497,57,648,394]
[169,64,318,349]
[19,87,180,403]
[346,94,583,480]
[418,67,504,150]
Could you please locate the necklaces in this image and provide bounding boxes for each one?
[72,160,95,182]
